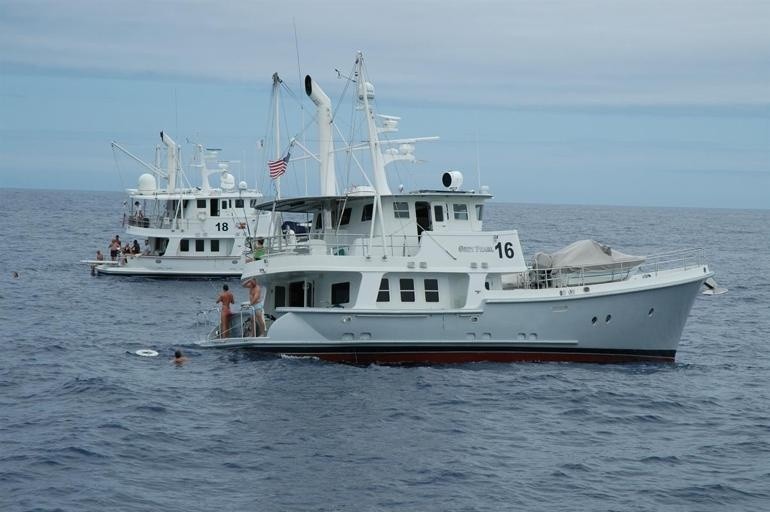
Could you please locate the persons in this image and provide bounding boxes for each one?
[254,237,266,258]
[216,284,234,338]
[90,235,152,272]
[134,201,143,227]
[285,225,297,252]
[174,350,181,358]
[241,277,265,337]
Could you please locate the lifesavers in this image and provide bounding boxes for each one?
[196,210,208,221]
[135,350,159,357]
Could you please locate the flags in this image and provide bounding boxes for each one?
[267,141,295,180]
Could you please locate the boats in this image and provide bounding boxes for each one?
[79,130,281,278]
[194,74,729,364]
